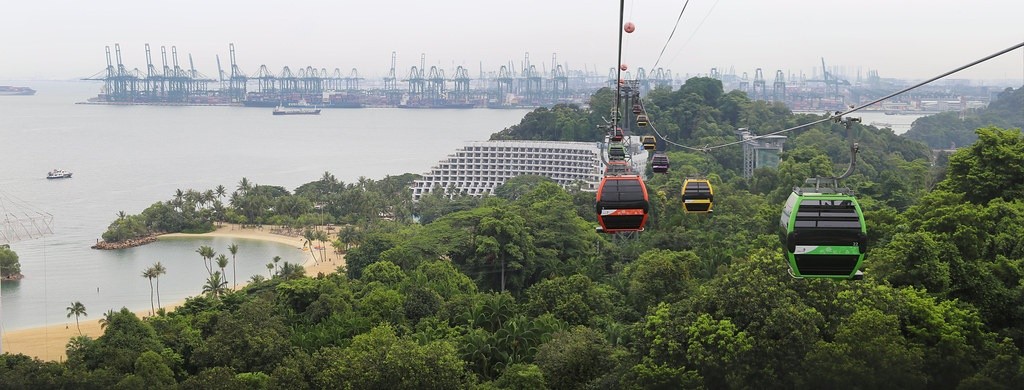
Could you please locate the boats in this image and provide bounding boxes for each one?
[46,169,74,180]
[0,85,36,97]
[273,104,322,115]
